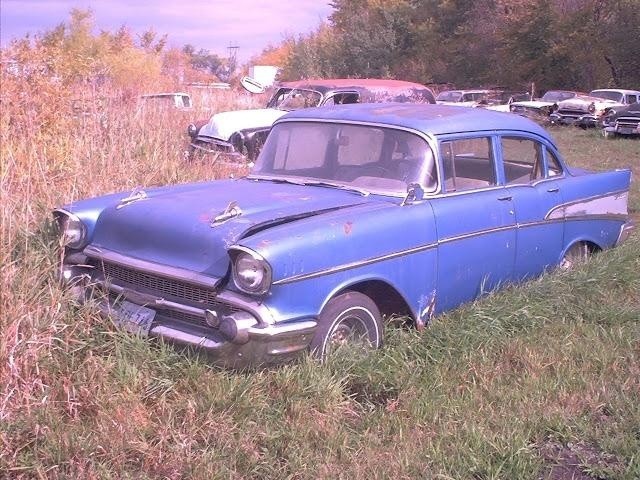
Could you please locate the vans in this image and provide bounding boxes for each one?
[139,92,193,112]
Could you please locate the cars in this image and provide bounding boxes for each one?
[438,86,640,136]
[186,81,436,168]
[51,102,631,374]
